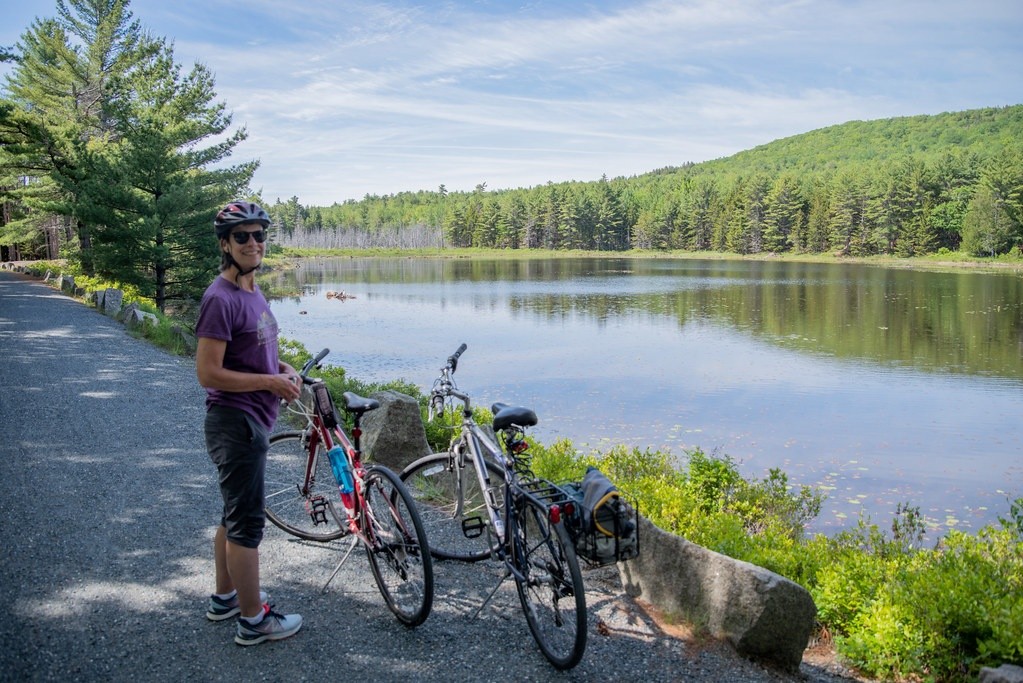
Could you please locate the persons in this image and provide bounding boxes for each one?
[192,200,304,645]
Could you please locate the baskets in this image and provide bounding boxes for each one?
[554,470,640,569]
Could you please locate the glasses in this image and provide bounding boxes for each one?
[230,229,268,245]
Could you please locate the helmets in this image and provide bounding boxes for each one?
[214,201,271,233]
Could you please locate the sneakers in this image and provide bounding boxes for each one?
[235,604,303,646]
[206,591,268,621]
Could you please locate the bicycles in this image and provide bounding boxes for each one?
[262,348,435,627]
[390,342,640,670]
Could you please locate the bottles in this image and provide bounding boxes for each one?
[327,446,355,495]
